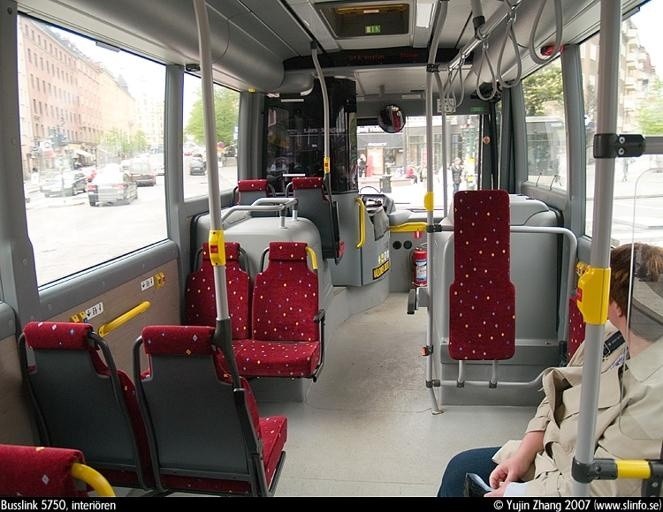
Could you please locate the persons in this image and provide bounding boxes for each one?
[436,242,663,497]
[406,166,418,184]
[358,158,366,178]
[451,158,462,198]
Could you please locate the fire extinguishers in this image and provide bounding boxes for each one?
[409,243,428,289]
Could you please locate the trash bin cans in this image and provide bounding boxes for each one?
[379,175,392,193]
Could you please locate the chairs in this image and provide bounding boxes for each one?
[0,174,346,497]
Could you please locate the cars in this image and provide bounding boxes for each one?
[184,149,205,176]
[121,157,164,187]
[87,164,138,208]
[39,170,86,198]
